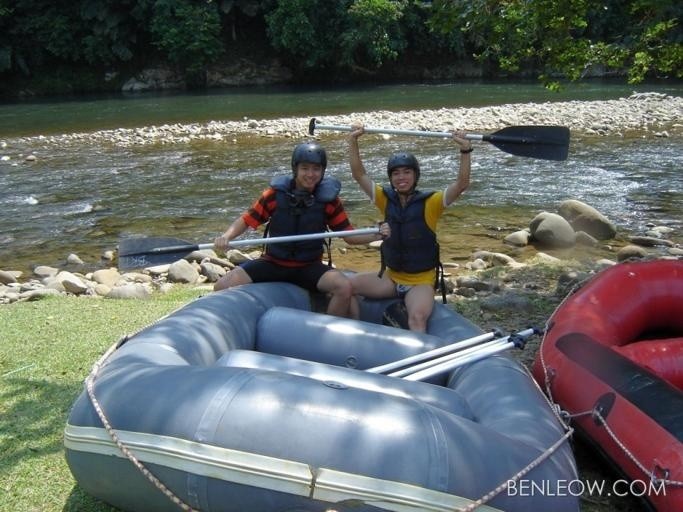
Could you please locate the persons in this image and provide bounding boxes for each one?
[348,121,472,335]
[211,141,392,318]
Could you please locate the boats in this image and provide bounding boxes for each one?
[63,267,579,512]
[533,261,683,510]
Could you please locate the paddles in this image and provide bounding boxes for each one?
[118,222,388,273]
[309,119,569,160]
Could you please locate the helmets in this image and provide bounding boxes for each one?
[291,141,328,185]
[387,152,420,189]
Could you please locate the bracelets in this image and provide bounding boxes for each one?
[459,145,473,154]
